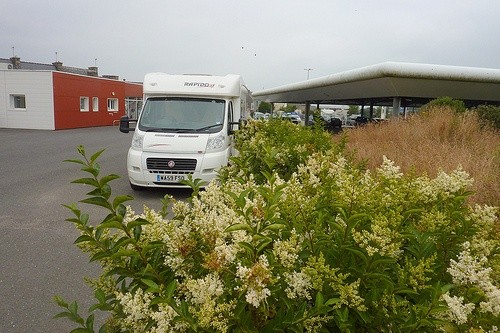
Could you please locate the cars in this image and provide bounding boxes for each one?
[252,110,408,129]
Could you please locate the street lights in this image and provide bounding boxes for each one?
[56,52,57,61]
[95,58,97,66]
[303,67,313,79]
[12,46,14,56]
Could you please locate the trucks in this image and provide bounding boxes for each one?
[118,72,247,194]
